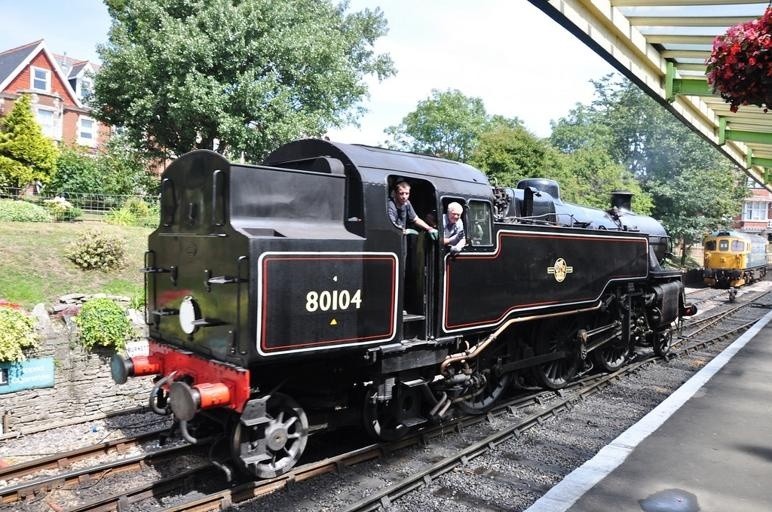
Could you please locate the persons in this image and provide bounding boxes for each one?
[441,202,464,256]
[388,182,438,243]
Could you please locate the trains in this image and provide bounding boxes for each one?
[702,222,771,290]
[107,133,704,494]
